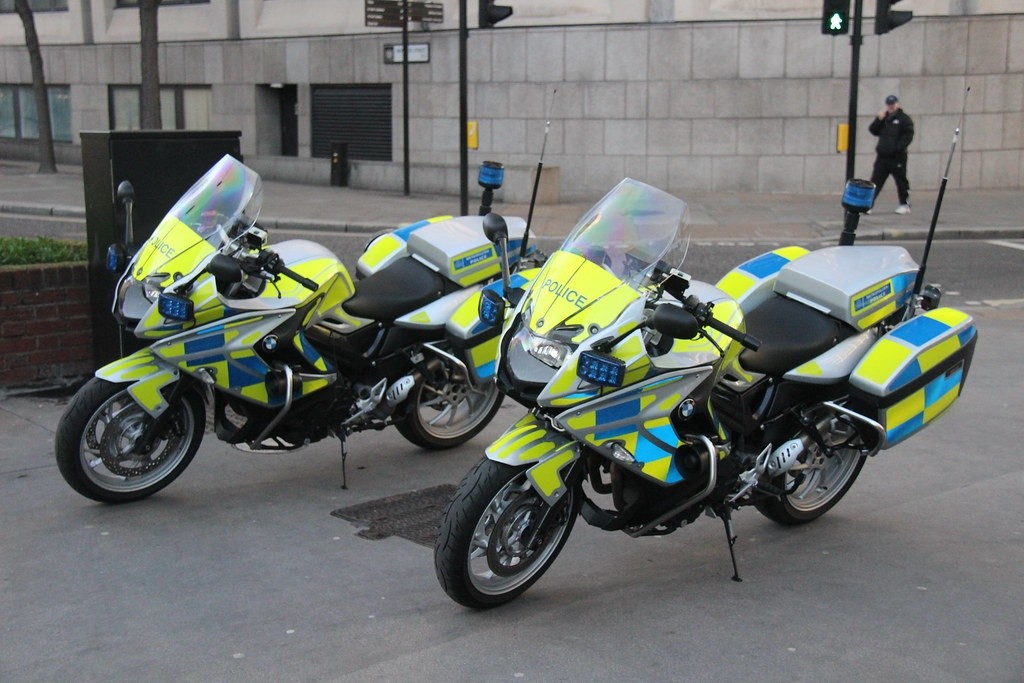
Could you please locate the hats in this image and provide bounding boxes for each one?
[886,95,897,105]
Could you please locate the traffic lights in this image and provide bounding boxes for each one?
[821,1,851,34]
[478,1,511,30]
[874,1,914,36]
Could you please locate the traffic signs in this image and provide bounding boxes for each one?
[364,0,443,31]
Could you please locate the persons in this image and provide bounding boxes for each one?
[867,96,914,215]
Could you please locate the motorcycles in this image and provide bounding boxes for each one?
[54,152,553,509]
[431,175,982,620]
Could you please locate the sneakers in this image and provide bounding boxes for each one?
[864,210,873,215]
[894,205,911,214]
